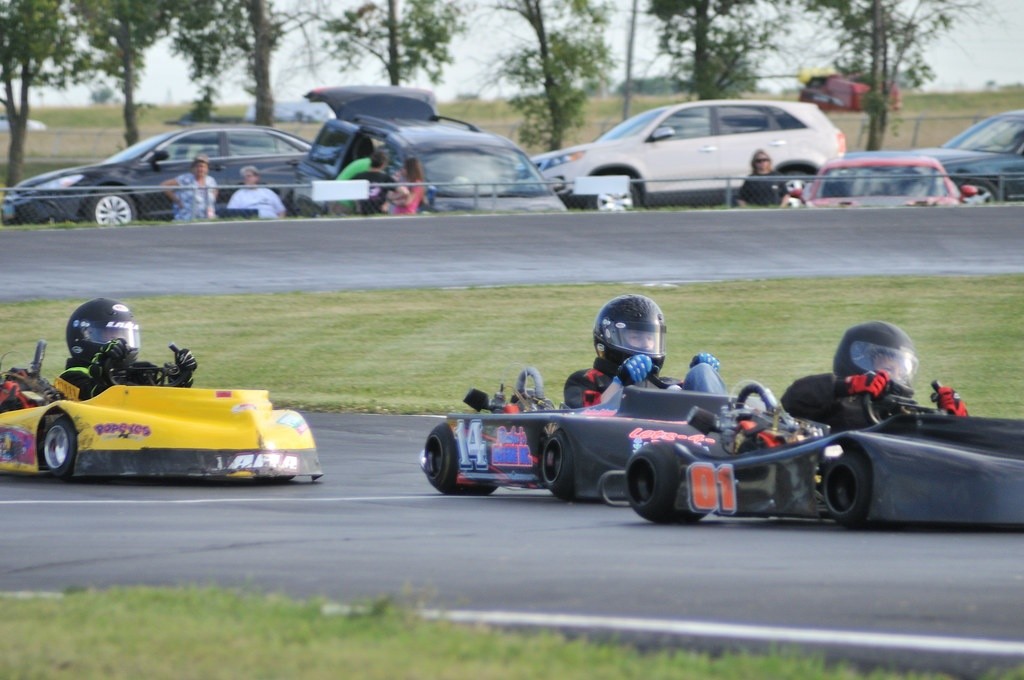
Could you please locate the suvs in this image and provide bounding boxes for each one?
[509,98,846,211]
[294,85,570,220]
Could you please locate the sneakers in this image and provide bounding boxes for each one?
[931,379,969,418]
[845,372,889,397]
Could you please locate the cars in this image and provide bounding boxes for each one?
[0,124,315,228]
[780,153,979,208]
[909,109,1024,205]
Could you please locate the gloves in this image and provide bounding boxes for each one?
[89,338,138,385]
[617,354,651,382]
[689,352,720,370]
[156,343,198,388]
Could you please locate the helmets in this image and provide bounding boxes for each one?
[592,294,666,373]
[67,299,138,361]
[834,321,916,398]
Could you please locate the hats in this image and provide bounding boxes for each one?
[194,154,209,163]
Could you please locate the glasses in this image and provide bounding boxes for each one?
[755,159,770,163]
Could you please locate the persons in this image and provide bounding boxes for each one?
[782,320,969,435]
[732,151,790,207]
[393,158,423,215]
[160,154,219,221]
[351,151,410,216]
[58,297,198,403]
[226,166,287,217]
[563,295,720,409]
